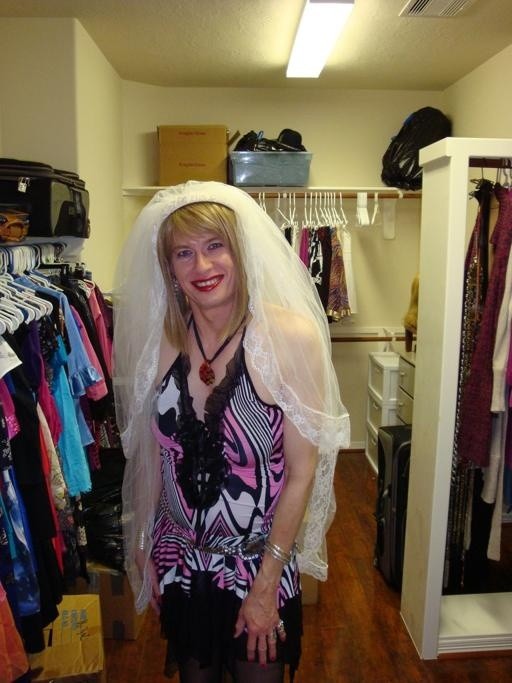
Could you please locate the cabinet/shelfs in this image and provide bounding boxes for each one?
[121,186,422,342]
[397,352,415,427]
[365,352,398,478]
[400,137,511,661]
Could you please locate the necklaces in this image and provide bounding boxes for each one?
[192,312,248,386]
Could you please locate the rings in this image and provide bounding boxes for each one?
[277,620,285,633]
[269,628,277,640]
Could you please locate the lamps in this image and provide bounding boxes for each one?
[285,0,355,79]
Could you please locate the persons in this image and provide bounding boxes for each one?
[109,178,352,680]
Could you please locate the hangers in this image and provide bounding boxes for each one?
[0,240,95,338]
[257,192,378,234]
[469,158,512,196]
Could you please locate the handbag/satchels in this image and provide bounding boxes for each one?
[380,106,451,192]
[0,209,30,242]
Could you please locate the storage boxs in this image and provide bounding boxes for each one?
[87,560,148,641]
[157,124,240,186]
[28,594,106,683]
[228,150,312,186]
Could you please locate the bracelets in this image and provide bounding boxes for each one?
[265,541,291,565]
[135,519,149,552]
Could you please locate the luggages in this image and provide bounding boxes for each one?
[373,424,412,593]
[0,158,91,238]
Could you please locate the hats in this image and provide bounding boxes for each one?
[262,128,304,151]
